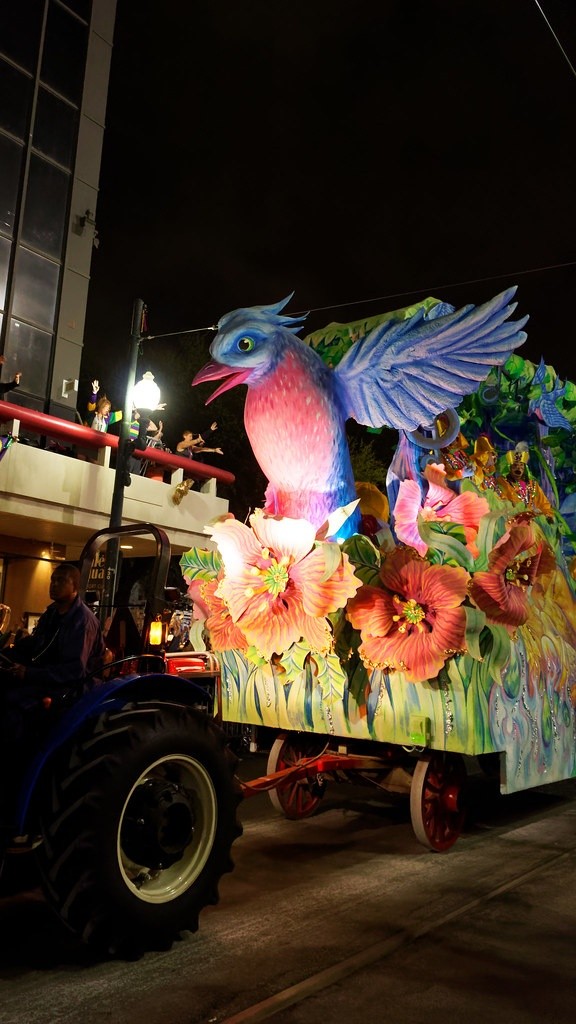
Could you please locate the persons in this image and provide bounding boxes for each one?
[77,379,223,482]
[0,563,105,854]
[0,356,22,429]
[431,421,555,525]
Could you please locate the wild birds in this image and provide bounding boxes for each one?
[192,283,529,547]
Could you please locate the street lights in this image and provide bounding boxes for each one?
[100,370,161,629]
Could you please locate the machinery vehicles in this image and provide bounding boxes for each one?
[0,522,242,959]
